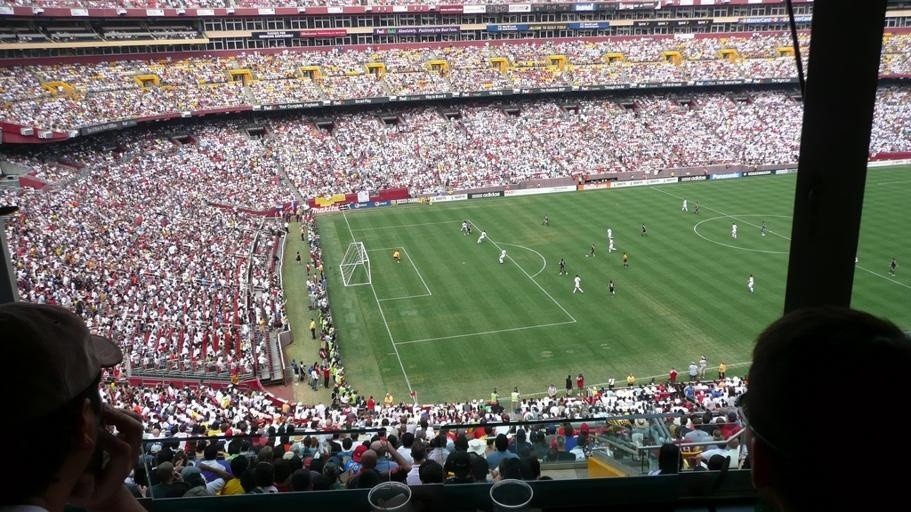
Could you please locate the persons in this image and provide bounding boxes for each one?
[478,229,488,244]
[760,220,769,237]
[731,223,738,239]
[499,248,507,264]
[393,249,401,264]
[345,354,752,488]
[585,242,597,258]
[559,258,569,276]
[2,1,345,512]
[607,228,615,242]
[623,252,629,269]
[542,212,551,226]
[747,274,755,293]
[640,224,649,237]
[608,280,616,296]
[573,275,584,294]
[608,238,617,254]
[888,257,898,277]
[2,302,147,510]
[731,305,911,511]
[461,220,469,232]
[693,200,700,215]
[465,222,472,236]
[681,197,689,215]
[346,0,911,205]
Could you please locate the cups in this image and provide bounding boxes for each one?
[491,478,535,510]
[367,481,414,511]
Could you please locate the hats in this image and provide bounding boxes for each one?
[292,435,307,442]
[0,299,127,416]
[180,465,202,479]
[150,444,163,453]
[322,440,385,482]
